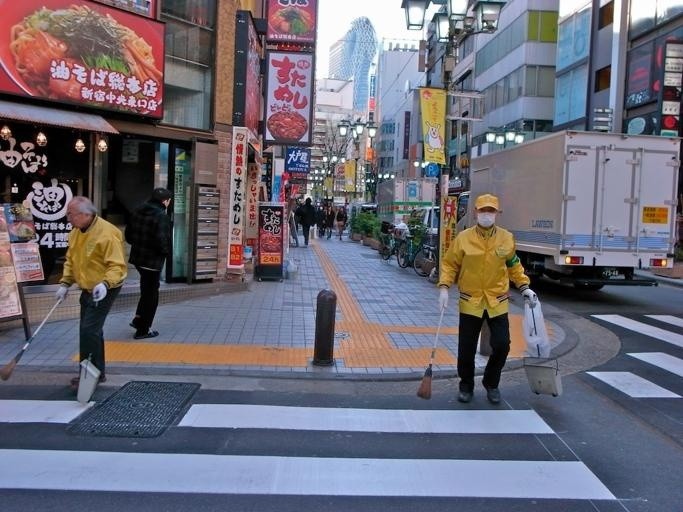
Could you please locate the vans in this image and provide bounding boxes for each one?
[408,205,440,234]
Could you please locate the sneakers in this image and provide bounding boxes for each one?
[458,392,472,402]
[71,370,106,384]
[482,378,500,403]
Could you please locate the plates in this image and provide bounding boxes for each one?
[0,59,163,116]
[261,237,281,253]
[268,4,315,36]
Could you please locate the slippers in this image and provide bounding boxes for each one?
[129,323,159,339]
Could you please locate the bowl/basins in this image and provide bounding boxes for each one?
[6,223,34,237]
[266,111,308,142]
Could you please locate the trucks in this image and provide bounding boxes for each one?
[376,177,437,226]
[458,130,680,293]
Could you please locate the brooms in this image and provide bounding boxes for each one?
[0,289,70,383]
[416,307,446,401]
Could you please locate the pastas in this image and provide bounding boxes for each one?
[10,6,163,113]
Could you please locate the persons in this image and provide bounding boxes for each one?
[56,197,127,386]
[125,189,174,338]
[294,197,348,248]
[437,195,538,406]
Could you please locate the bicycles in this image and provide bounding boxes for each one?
[380,223,411,269]
[413,234,439,276]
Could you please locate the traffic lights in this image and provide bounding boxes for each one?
[663,115,678,129]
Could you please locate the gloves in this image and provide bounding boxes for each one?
[92,283,107,302]
[439,288,448,311]
[56,287,68,304]
[521,289,537,304]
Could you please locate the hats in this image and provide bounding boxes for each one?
[475,194,499,211]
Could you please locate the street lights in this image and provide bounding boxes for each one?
[410,160,432,177]
[401,0,505,199]
[310,153,395,207]
[482,131,525,150]
[337,117,377,199]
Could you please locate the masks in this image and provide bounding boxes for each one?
[477,212,495,227]
